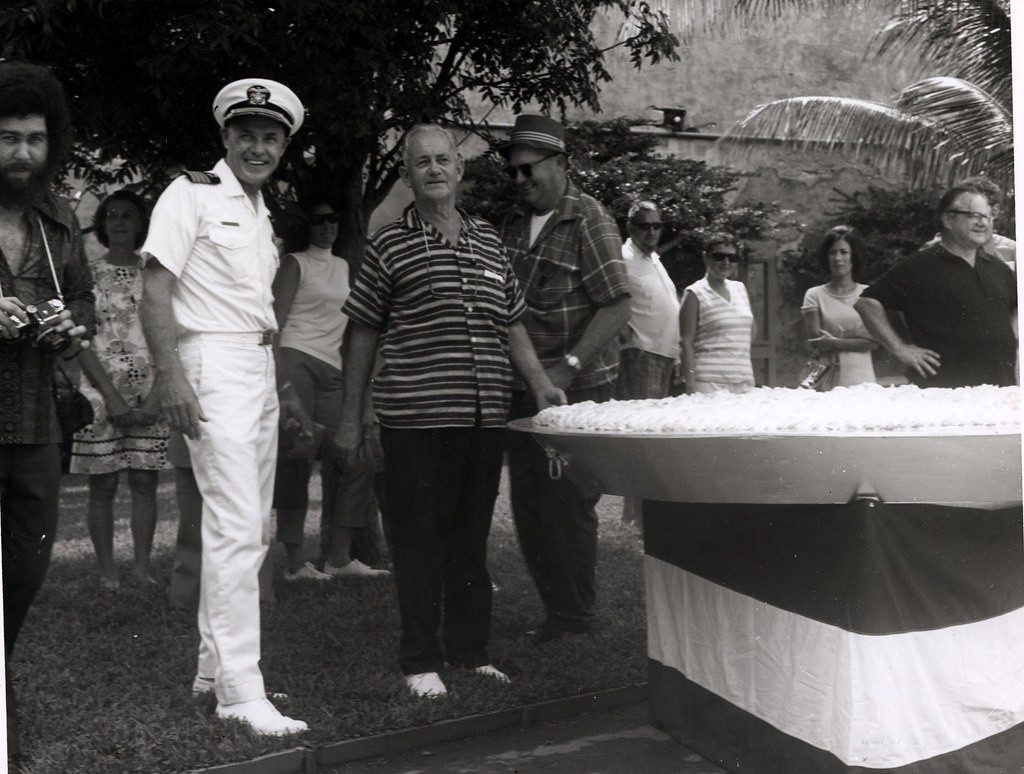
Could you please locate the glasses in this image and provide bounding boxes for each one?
[709,251,743,262]
[506,151,558,178]
[945,209,994,228]
[309,212,340,224]
[631,222,663,230]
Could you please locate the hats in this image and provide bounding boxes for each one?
[499,115,572,156]
[212,78,304,137]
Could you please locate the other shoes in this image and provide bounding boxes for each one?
[169,608,190,631]
[526,614,593,637]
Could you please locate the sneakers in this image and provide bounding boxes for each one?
[217,699,307,736]
[404,671,447,696]
[444,660,513,685]
[282,562,335,581]
[192,675,289,698]
[323,558,390,576]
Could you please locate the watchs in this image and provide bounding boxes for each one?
[564,354,583,372]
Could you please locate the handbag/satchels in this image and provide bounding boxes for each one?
[51,355,94,438]
[801,355,831,390]
[671,377,687,396]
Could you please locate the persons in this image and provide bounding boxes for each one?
[70,190,173,594]
[331,119,569,701]
[140,77,309,736]
[270,191,391,581]
[619,200,680,530]
[801,225,879,391]
[851,185,1020,388]
[481,114,633,635]
[679,234,757,392]
[0,63,97,704]
[917,177,1017,271]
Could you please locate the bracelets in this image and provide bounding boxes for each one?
[684,369,696,376]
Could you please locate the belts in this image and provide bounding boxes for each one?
[181,331,273,345]
